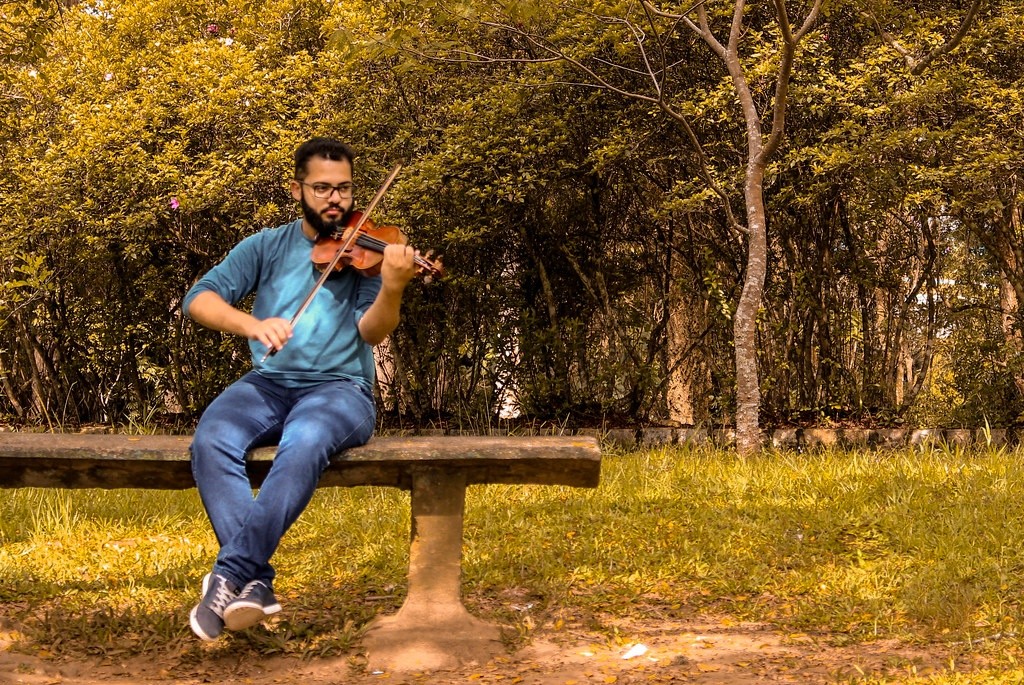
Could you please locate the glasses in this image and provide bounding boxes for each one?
[297,180,358,199]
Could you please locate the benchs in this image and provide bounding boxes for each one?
[0,431,603,615]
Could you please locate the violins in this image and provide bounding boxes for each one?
[309,210,443,284]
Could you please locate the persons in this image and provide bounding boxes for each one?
[182,137,416,642]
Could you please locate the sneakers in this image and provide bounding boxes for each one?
[189,571,282,641]
